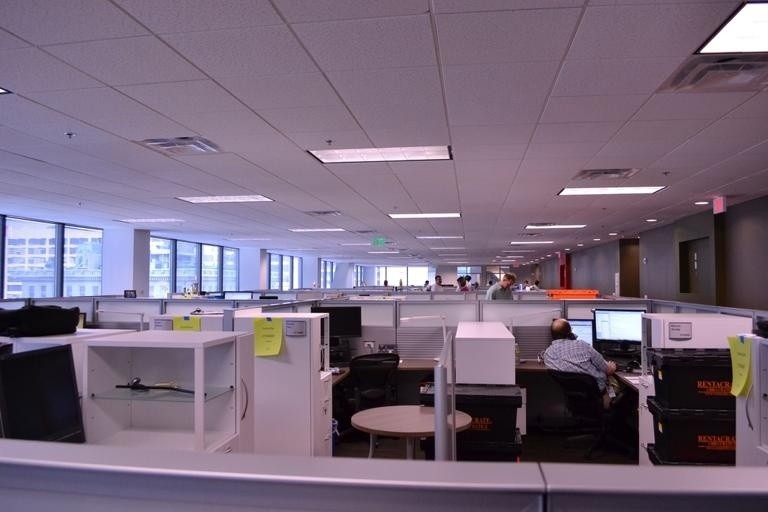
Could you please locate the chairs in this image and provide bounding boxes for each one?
[548,369,629,460]
[339,353,399,447]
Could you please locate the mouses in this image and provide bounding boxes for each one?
[626,366,633,371]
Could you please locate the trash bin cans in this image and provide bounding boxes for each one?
[332,418,338,456]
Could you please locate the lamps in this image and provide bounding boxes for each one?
[96,309,144,330]
[509,308,563,334]
[400,315,446,345]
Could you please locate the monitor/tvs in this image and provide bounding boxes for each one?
[594,309,646,352]
[311,306,361,349]
[260,296,278,299]
[124,290,136,298]
[0,343,87,445]
[76,313,87,328]
[552,318,594,348]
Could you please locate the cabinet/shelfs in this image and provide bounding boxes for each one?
[83,330,240,453]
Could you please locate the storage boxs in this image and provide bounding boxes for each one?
[646,347,736,465]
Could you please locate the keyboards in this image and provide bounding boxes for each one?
[331,362,350,368]
[606,358,631,371]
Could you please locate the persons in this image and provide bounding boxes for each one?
[543,319,624,409]
[424,280,432,291]
[486,271,517,300]
[464,275,540,291]
[456,276,470,291]
[423,274,445,292]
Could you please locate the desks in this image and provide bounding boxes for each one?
[350,412,489,459]
[516,360,639,393]
[333,358,437,388]
